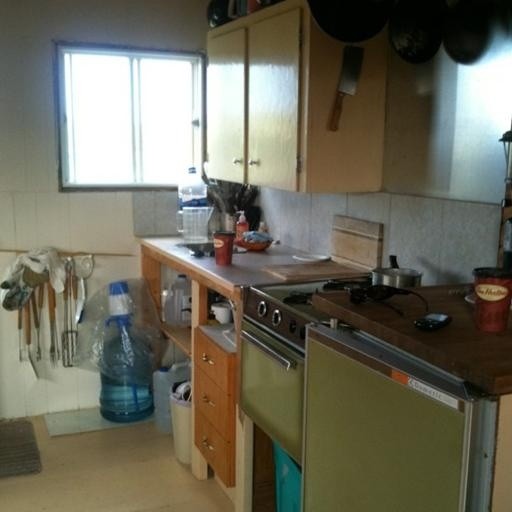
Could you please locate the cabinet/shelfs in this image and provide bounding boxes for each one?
[201,0,392,195]
[194,323,243,489]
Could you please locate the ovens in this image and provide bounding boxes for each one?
[238,318,305,466]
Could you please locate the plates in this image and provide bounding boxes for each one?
[291,252,331,263]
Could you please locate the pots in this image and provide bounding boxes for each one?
[371,254,424,289]
[306,0,489,65]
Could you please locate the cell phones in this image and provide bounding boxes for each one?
[413,312,452,332]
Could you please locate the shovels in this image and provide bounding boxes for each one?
[22,298,40,380]
[61,274,83,367]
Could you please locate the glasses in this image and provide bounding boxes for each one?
[347,283,429,316]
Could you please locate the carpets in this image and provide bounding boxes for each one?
[0,418,42,479]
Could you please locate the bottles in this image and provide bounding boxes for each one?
[178,166,209,210]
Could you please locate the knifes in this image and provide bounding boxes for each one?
[326,46,366,132]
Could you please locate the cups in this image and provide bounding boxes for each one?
[176,206,215,244]
[212,302,232,327]
[212,230,236,267]
[471,266,511,336]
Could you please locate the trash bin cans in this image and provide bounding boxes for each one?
[168,382,193,466]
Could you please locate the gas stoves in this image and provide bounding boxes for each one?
[248,276,381,324]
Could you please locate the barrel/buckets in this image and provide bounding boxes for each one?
[165,274,192,328]
[100,279,154,423]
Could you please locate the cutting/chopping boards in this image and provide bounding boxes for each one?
[259,259,371,283]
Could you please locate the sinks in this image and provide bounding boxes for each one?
[174,242,248,255]
